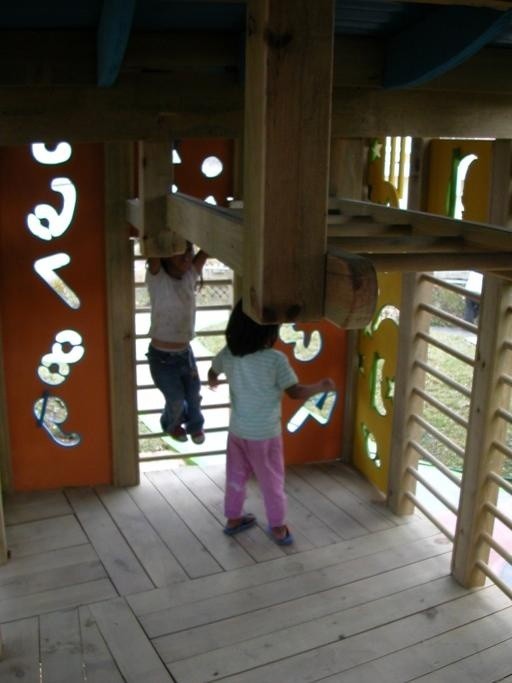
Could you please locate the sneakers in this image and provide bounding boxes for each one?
[168,426,188,442]
[191,429,204,444]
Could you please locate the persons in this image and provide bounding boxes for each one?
[207,298,336,546]
[145,239,208,444]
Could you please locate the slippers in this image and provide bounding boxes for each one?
[271,524,293,545]
[223,512,256,535]
